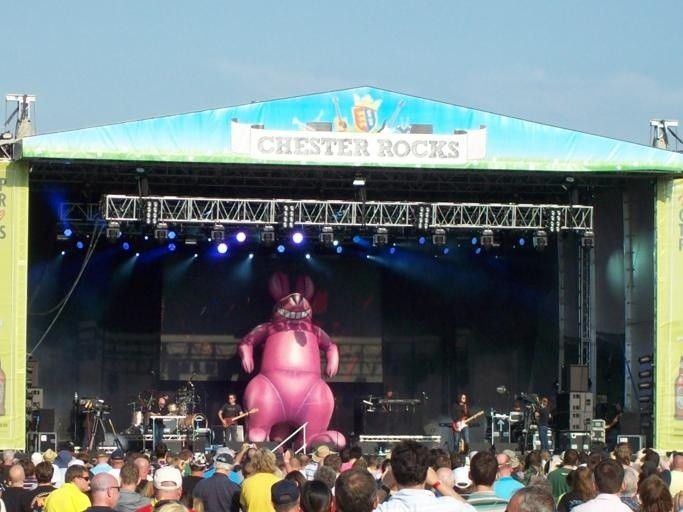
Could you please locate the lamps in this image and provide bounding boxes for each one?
[104,194,594,256]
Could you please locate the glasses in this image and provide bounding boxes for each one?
[72,475,89,481]
[111,487,121,491]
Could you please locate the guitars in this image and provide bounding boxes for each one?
[223,408,259,428]
[453,411,485,432]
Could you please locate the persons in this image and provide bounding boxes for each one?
[149,398,169,445]
[218,394,248,442]
[535,398,551,450]
[595,393,623,447]
[452,393,472,451]
[0,440,683,512]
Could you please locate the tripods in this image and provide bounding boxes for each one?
[520,406,540,454]
[87,414,126,457]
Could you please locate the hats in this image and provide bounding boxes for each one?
[111,450,124,460]
[272,480,299,504]
[503,449,520,469]
[154,467,182,490]
[454,467,473,490]
[217,453,234,465]
[43,449,58,461]
[312,445,332,463]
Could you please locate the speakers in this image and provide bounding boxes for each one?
[224,441,281,454]
[310,441,334,452]
[465,442,492,454]
[554,393,592,431]
[616,434,645,453]
[493,442,521,455]
[561,365,589,392]
[32,409,55,432]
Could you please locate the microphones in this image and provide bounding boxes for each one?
[188,373,195,385]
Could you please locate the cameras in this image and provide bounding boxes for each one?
[247,445,253,449]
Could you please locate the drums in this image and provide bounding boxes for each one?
[131,411,143,426]
[167,403,177,412]
[144,412,160,426]
[187,413,208,428]
